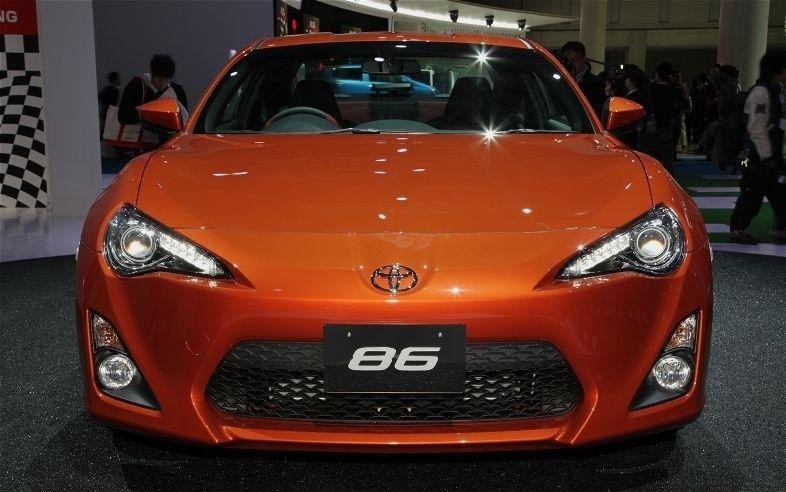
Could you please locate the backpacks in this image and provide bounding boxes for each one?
[726,81,777,144]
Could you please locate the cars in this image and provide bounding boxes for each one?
[76,29,713,470]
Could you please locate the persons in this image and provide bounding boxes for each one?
[277,5,287,36]
[118,54,188,176]
[100,71,121,144]
[305,19,317,35]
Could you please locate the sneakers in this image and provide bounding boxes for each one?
[673,136,712,163]
[728,229,761,245]
[770,227,786,245]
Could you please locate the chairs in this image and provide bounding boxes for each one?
[427,76,521,132]
[282,79,353,130]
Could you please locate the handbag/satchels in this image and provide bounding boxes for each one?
[102,75,161,151]
